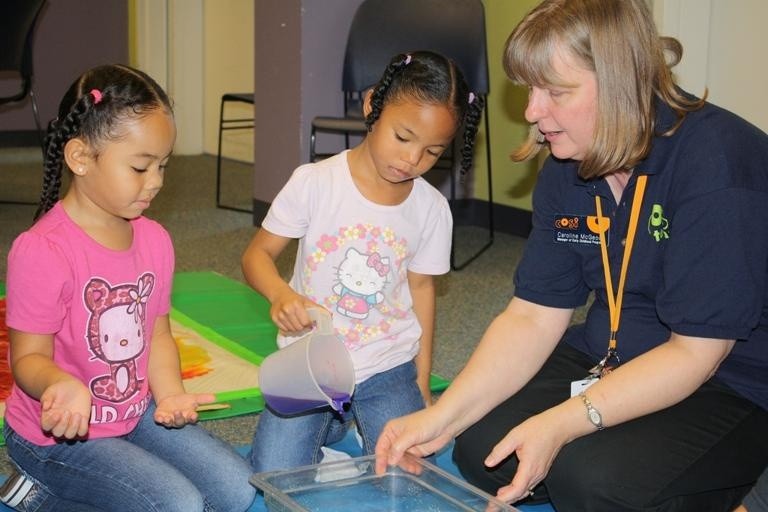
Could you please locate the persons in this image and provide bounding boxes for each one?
[375,0,768,512]
[0,65,259,512]
[240,51,485,494]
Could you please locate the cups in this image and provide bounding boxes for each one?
[259,307,356,416]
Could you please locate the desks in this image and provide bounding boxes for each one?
[214,93,256,208]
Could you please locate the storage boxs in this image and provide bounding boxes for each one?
[250,450,521,512]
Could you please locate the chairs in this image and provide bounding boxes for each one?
[0,0,59,205]
[308,0,496,271]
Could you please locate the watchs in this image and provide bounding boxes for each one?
[577,390,605,433]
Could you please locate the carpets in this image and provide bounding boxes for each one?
[0,429,556,512]
[0,270,455,445]
